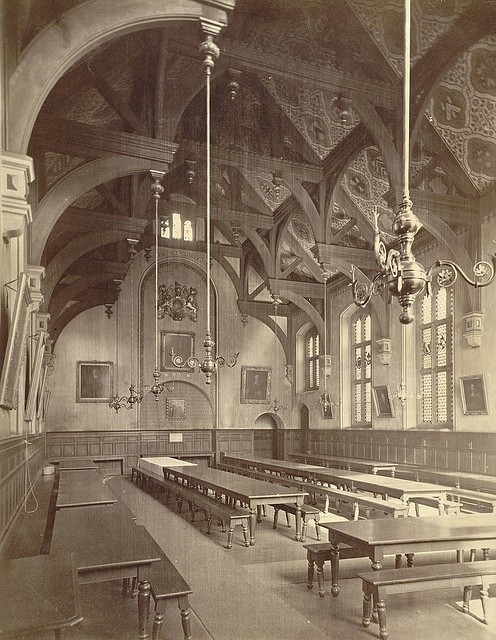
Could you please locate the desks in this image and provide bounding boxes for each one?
[223,454,286,473]
[55,469,118,507]
[138,456,197,479]
[415,466,495,493]
[0,551,85,640]
[316,513,496,597]
[261,462,454,517]
[48,505,160,640]
[161,464,308,546]
[58,458,97,470]
[288,453,396,478]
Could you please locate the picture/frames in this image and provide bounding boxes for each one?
[457,373,489,416]
[370,385,394,419]
[75,360,116,404]
[0,274,34,410]
[319,394,333,419]
[160,330,194,373]
[26,330,52,420]
[165,397,186,423]
[240,365,271,404]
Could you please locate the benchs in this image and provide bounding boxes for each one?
[411,494,463,516]
[355,559,496,640]
[303,542,403,599]
[215,463,233,473]
[104,482,137,521]
[454,486,495,512]
[280,477,409,522]
[130,466,154,492]
[236,465,278,486]
[149,475,250,550]
[95,466,106,478]
[270,502,321,544]
[129,525,194,639]
